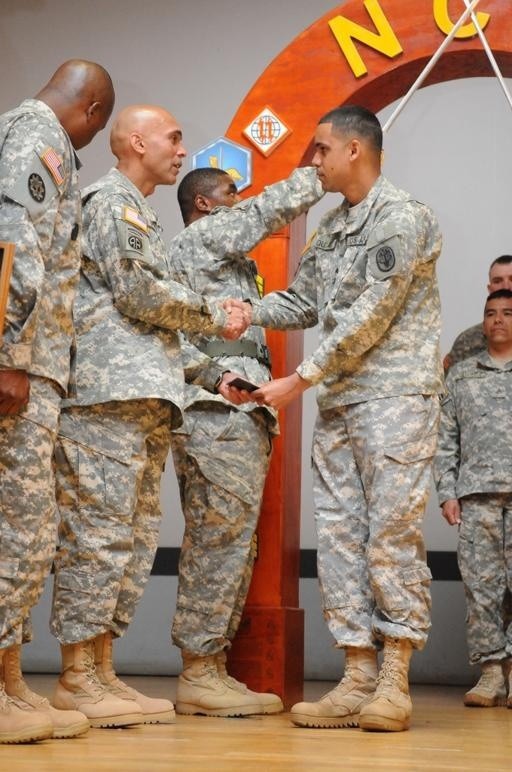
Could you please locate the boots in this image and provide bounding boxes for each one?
[0,642,88,746]
[290,637,415,732]
[52,631,178,728]
[177,651,285,719]
[464,656,512,711]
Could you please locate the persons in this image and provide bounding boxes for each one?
[49,103,258,728]
[164,165,327,717]
[1,61,116,745]
[441,255,511,378]
[428,288,512,710]
[221,104,447,732]
